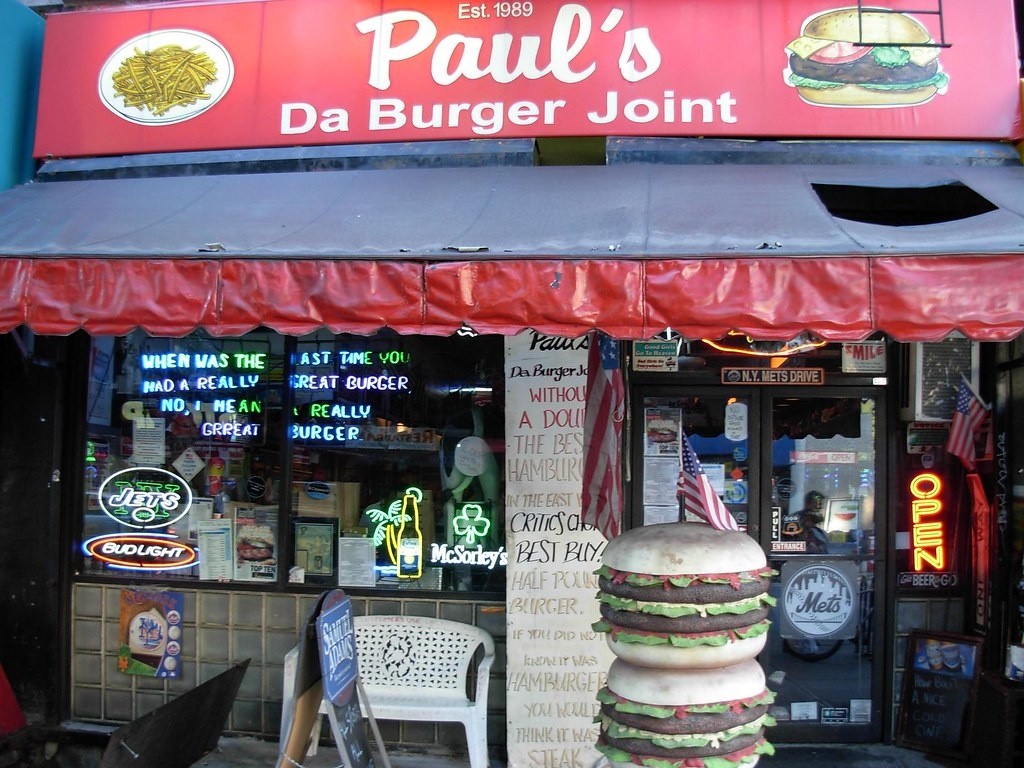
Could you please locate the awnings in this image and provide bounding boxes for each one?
[0,164,1024,341]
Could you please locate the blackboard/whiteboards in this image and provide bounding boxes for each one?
[326,684,396,768]
[896,628,982,760]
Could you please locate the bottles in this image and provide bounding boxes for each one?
[925,638,963,674]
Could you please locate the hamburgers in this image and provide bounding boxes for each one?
[592,656,778,768]
[590,522,779,671]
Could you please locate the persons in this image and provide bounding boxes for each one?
[787,491,828,554]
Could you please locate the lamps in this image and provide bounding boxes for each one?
[533,137,608,166]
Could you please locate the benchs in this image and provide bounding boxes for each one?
[277,615,496,767]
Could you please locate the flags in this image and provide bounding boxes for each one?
[677,432,738,531]
[947,378,987,471]
[581,334,626,540]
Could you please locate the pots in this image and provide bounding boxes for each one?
[237,542,274,561]
